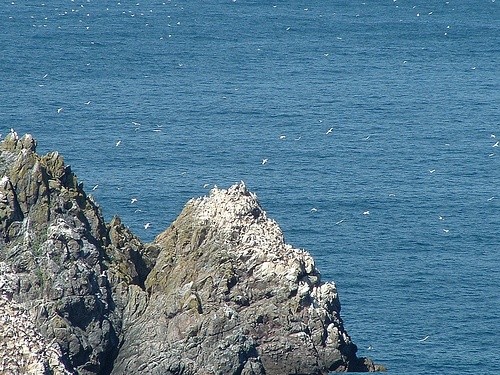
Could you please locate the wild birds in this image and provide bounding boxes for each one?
[130,198,138,204]
[443,228,450,233]
[428,169,435,174]
[0,1,454,147]
[92,185,99,191]
[262,159,268,165]
[492,142,499,148]
[491,133,496,138]
[336,220,343,225]
[144,222,151,229]
[363,211,370,215]
[310,208,318,212]
[204,184,210,189]
